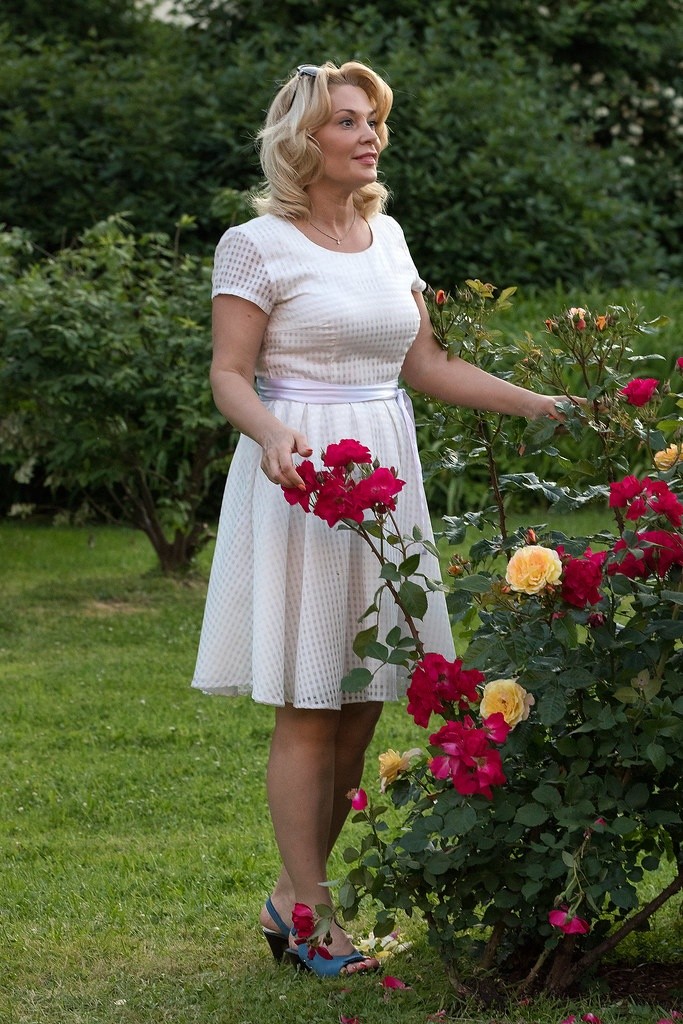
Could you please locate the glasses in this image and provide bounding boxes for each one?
[286,63,320,116]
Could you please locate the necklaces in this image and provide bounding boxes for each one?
[308,210,357,245]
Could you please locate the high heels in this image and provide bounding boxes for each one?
[285,925,383,981]
[262,898,292,966]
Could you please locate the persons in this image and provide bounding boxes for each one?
[193,64,601,976]
